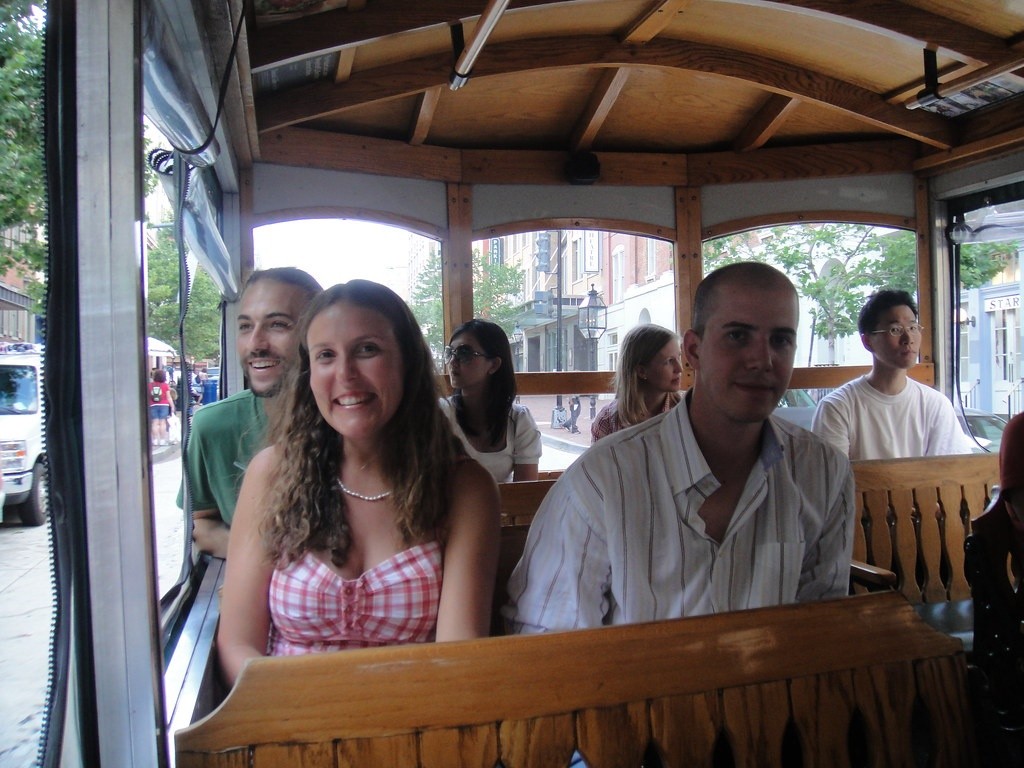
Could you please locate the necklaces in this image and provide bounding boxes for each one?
[337,476,394,500]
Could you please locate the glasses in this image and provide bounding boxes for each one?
[444,344,490,364]
[870,323,925,337]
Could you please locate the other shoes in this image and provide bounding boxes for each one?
[153,439,159,445]
[561,424,570,432]
[159,439,168,446]
[573,430,581,435]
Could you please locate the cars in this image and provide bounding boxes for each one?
[954,406,1008,453]
[207,367,220,378]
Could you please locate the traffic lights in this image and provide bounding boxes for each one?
[535,233,551,271]
[535,289,554,316]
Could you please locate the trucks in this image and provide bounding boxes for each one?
[577,282,608,420]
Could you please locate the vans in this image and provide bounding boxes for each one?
[0,343,48,527]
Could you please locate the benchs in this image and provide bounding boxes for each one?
[167,454,1001,768]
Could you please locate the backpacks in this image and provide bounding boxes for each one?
[150,381,164,403]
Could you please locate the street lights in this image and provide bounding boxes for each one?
[514,322,524,404]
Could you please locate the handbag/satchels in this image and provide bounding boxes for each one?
[167,413,183,443]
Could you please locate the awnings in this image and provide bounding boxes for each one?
[147,336,179,359]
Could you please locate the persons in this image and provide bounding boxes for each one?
[812,288,983,584]
[561,395,581,434]
[185,264,325,559]
[195,369,209,405]
[441,319,547,480]
[963,411,1024,768]
[586,326,690,451]
[498,262,859,768]
[217,282,500,691]
[190,371,205,416]
[145,371,178,447]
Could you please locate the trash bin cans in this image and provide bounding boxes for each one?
[201,379,218,405]
